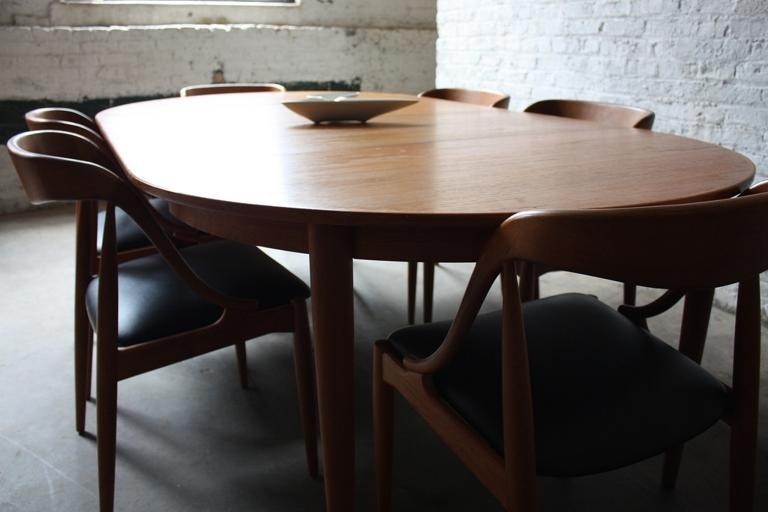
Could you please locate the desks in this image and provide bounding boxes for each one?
[96,90,756,512]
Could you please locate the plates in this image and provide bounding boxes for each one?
[282,92,421,127]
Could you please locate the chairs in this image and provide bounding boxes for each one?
[396,86,505,321]
[24,103,249,400]
[179,82,282,100]
[9,132,318,511]
[423,96,654,326]
[372,176,766,505]
